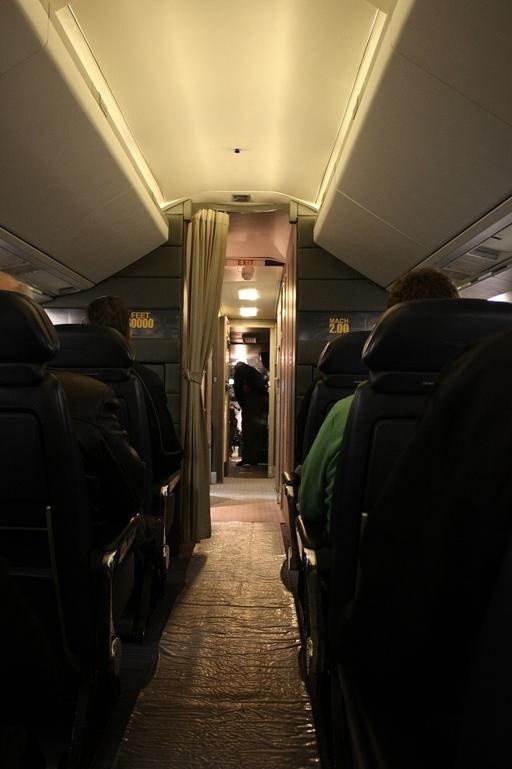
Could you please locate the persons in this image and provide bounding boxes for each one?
[231,359,268,469]
[82,296,183,461]
[291,269,463,541]
[1,270,143,519]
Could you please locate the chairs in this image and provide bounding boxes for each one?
[1,290,182,769]
[280,299,512,769]
